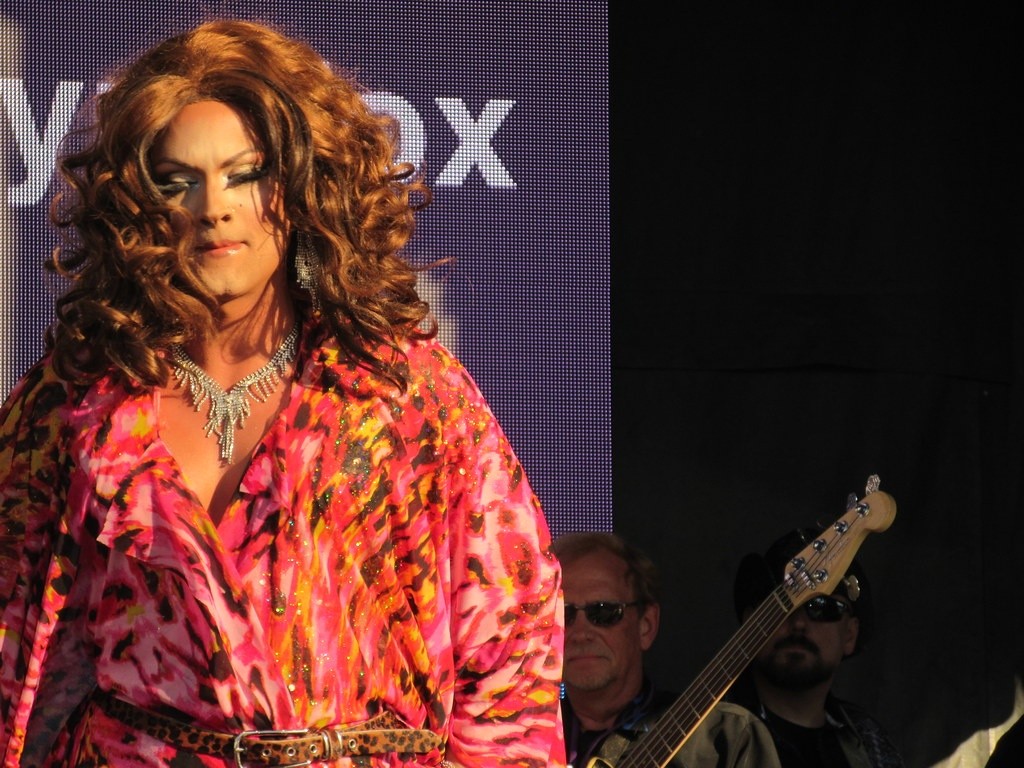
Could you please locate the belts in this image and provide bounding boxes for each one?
[92,690,442,768]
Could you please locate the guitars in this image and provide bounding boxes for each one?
[587,473,897,768]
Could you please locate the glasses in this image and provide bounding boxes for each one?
[565,602,642,627]
[804,595,860,623]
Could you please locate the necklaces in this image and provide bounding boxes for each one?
[162,322,301,465]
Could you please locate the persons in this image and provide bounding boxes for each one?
[734,537,906,768]
[551,535,781,768]
[1,18,567,768]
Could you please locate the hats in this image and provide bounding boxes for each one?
[731,529,884,628]
